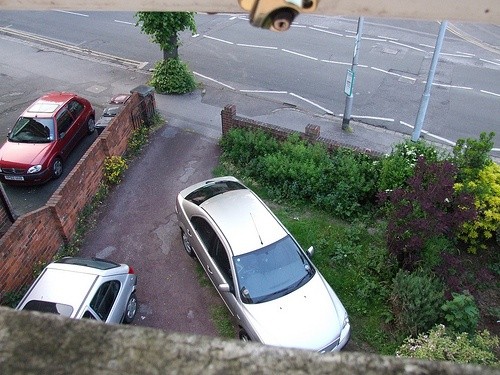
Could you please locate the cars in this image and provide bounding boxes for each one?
[15,254,141,325]
[0,91,95,187]
[176,175,353,355]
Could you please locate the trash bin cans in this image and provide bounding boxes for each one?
[102,104,122,116]
[130,84,156,119]
[108,93,131,105]
[93,117,114,135]
